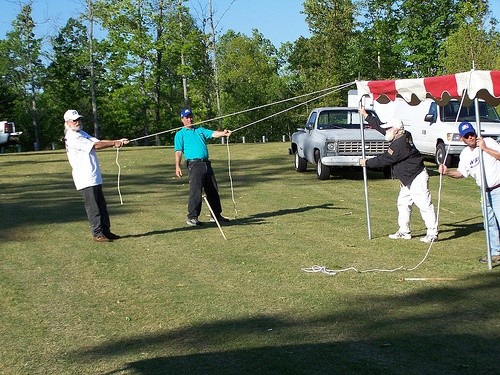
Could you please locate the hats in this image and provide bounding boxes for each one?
[459,122,475,136]
[379,119,404,130]
[181,108,193,117]
[64,109,84,121]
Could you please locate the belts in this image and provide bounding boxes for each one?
[188,158,208,162]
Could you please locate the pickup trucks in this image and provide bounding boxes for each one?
[288,106,394,181]
[0,120,23,145]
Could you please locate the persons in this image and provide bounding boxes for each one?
[63,109,129,242]
[439,120,500,263]
[174,109,232,228]
[358,104,438,243]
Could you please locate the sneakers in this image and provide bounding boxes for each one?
[420,235,438,243]
[388,230,411,240]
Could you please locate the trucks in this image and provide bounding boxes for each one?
[346,89,500,168]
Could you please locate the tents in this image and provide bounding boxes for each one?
[354,69,500,270]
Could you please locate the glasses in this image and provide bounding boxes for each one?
[463,132,474,139]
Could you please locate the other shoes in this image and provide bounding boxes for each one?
[93,230,120,242]
[210,216,229,222]
[479,253,500,263]
[186,218,203,227]
